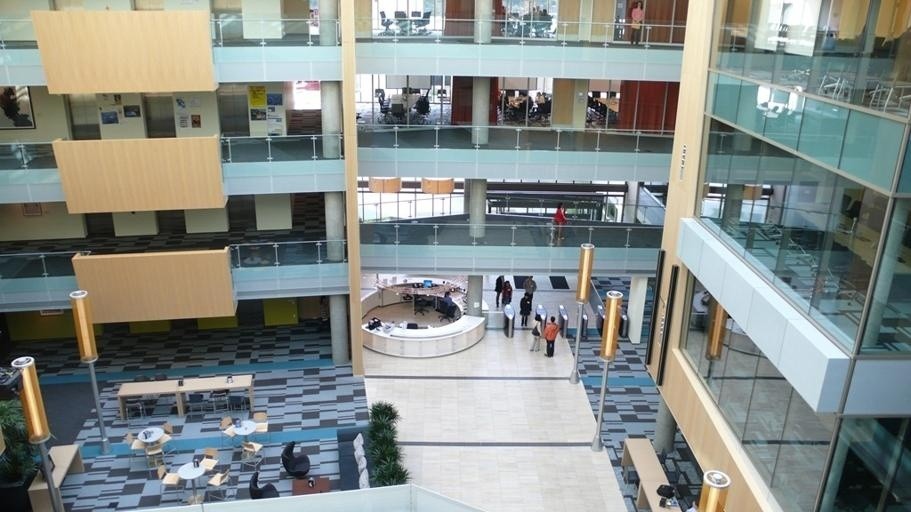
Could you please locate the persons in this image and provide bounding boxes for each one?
[532,6,548,31]
[0,88,20,121]
[495,275,504,307]
[632,2,645,45]
[444,292,457,317]
[502,280,512,306]
[529,314,541,351]
[520,293,532,326]
[555,202,568,240]
[498,89,547,112]
[523,276,536,310]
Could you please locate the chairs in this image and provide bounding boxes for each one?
[407,323,417,329]
[415,295,429,316]
[380,10,555,36]
[377,88,551,124]
[438,305,456,322]
[123,373,311,505]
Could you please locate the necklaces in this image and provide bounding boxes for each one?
[544,317,559,356]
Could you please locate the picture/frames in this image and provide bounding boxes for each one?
[1,86,37,130]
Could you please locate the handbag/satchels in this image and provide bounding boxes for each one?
[532,327,540,336]
[632,20,640,29]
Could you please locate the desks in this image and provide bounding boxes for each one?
[361,315,486,358]
[376,282,489,329]
[621,436,682,512]
[27,443,84,512]
